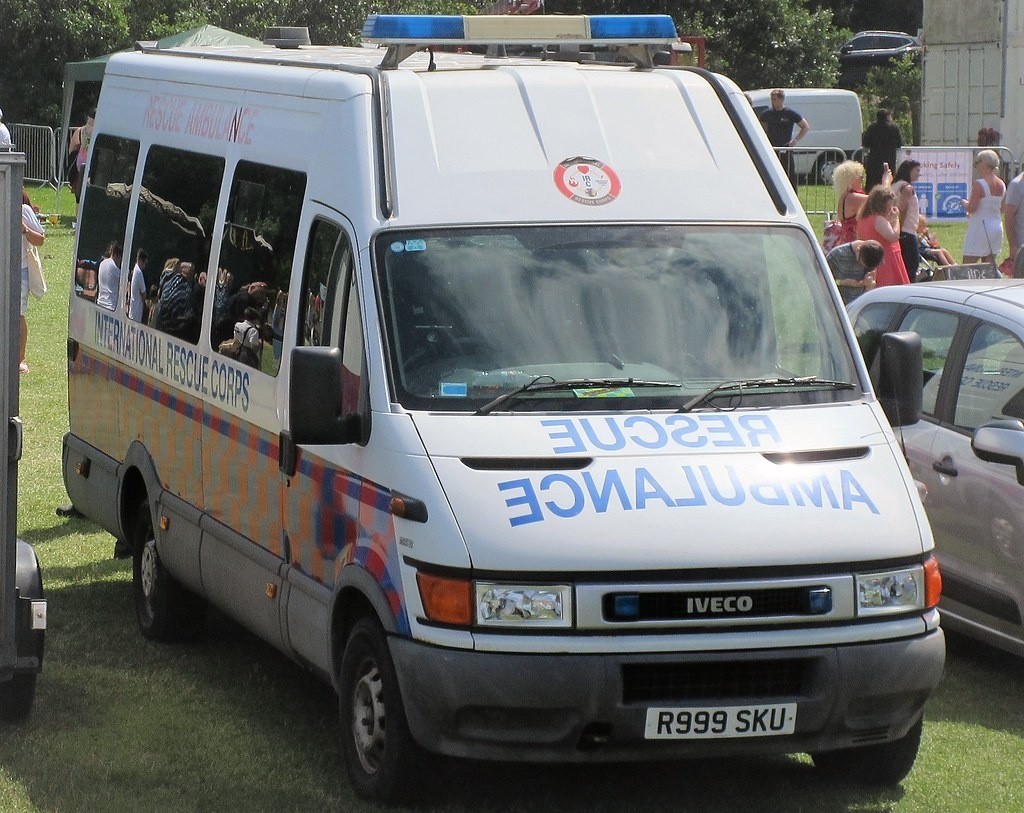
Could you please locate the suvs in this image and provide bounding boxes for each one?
[827,29,927,94]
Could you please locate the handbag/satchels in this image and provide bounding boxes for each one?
[218,339,260,369]
[27,247,47,300]
[822,220,842,252]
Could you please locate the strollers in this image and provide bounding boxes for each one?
[917,239,941,280]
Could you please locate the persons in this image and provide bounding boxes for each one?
[756,87,809,192]
[959,147,1006,265]
[94,244,273,369]
[857,187,908,287]
[924,217,957,266]
[858,106,903,193]
[898,160,922,282]
[21,188,51,375]
[1001,164,1022,276]
[830,157,894,244]
[821,238,884,302]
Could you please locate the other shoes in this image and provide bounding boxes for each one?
[19,362,29,374]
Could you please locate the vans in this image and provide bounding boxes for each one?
[742,84,865,187]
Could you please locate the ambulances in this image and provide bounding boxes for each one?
[55,8,948,792]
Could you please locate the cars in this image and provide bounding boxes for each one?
[842,276,1024,670]
